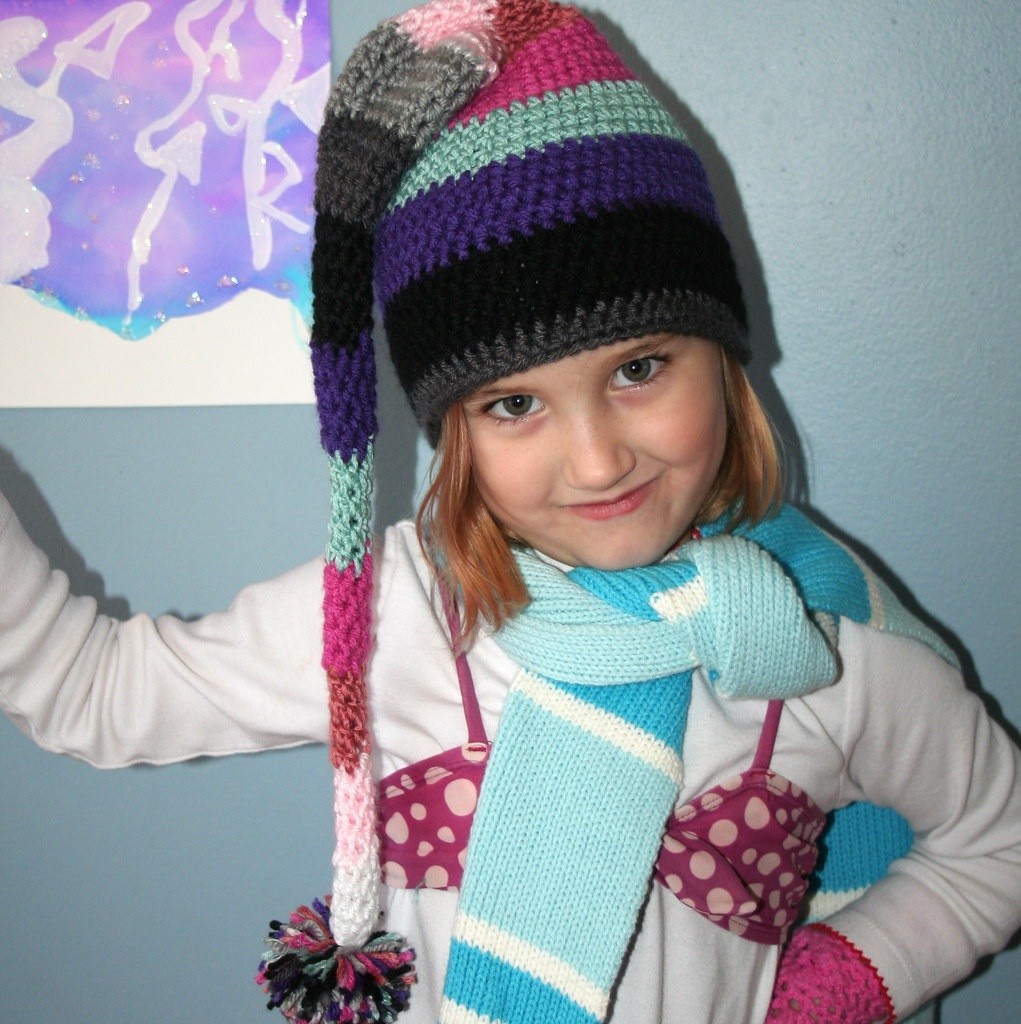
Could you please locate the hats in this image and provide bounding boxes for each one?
[261,0,751,1024]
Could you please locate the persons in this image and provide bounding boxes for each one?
[0,1,1020,1024]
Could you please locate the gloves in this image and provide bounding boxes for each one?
[763,922,892,1024]
